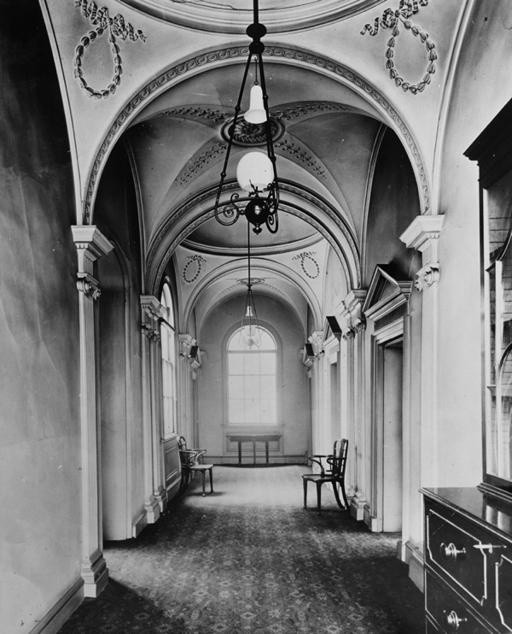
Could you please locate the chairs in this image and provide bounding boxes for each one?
[177,436,214,497]
[301,438,351,516]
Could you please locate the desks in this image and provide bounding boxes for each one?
[226,433,282,467]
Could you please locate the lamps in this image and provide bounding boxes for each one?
[237,223,264,351]
[213,0,285,233]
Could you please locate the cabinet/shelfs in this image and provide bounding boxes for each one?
[422,486,512,633]
[462,98,511,506]
